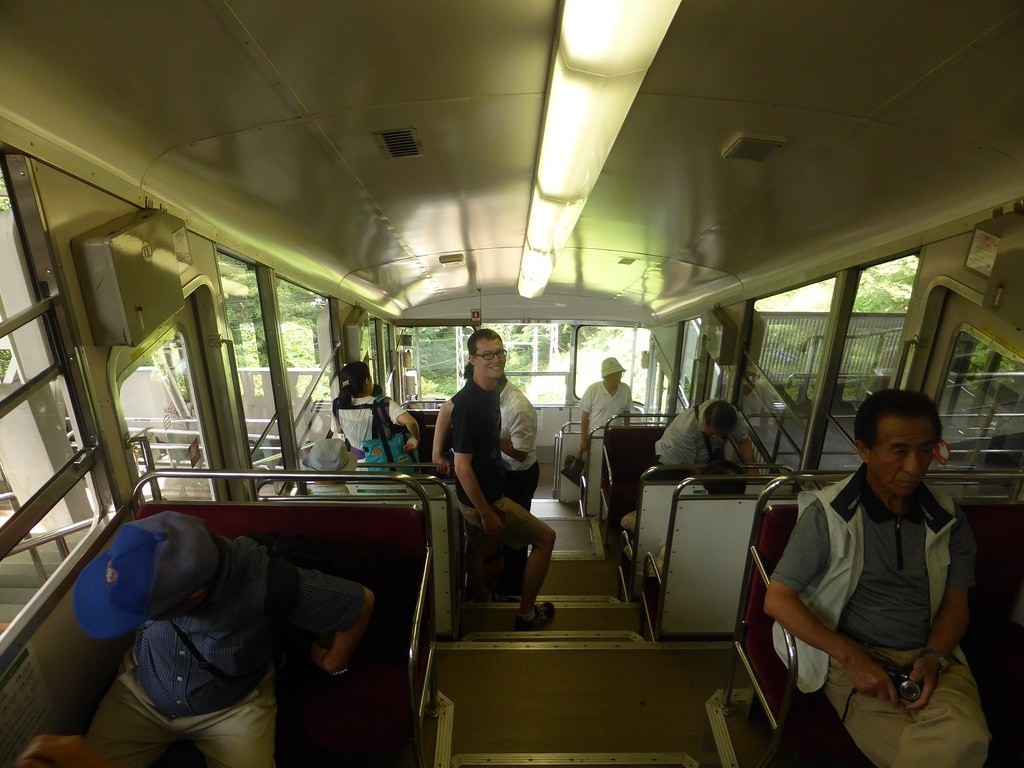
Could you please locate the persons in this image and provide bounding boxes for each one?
[621,397,760,592]
[578,357,632,450]
[71,510,374,768]
[431,329,555,632]
[763,388,993,768]
[301,438,357,497]
[464,362,540,561]
[331,360,421,473]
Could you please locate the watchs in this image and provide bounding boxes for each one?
[919,648,949,674]
[321,654,348,676]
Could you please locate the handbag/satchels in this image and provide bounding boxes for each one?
[359,432,420,474]
[708,446,728,474]
[558,448,587,489]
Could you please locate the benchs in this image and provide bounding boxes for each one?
[721,467,1024,768]
[598,414,726,548]
[254,478,468,640]
[296,481,469,611]
[132,470,437,768]
[641,477,819,643]
[619,464,792,603]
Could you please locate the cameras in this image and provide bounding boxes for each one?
[885,666,925,703]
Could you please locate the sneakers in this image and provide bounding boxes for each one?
[514,602,555,632]
[490,593,520,603]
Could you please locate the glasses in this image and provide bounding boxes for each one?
[473,349,507,360]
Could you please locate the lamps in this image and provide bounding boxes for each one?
[517,0,686,300]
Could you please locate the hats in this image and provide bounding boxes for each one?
[302,439,357,471]
[601,357,626,378]
[71,510,220,640]
